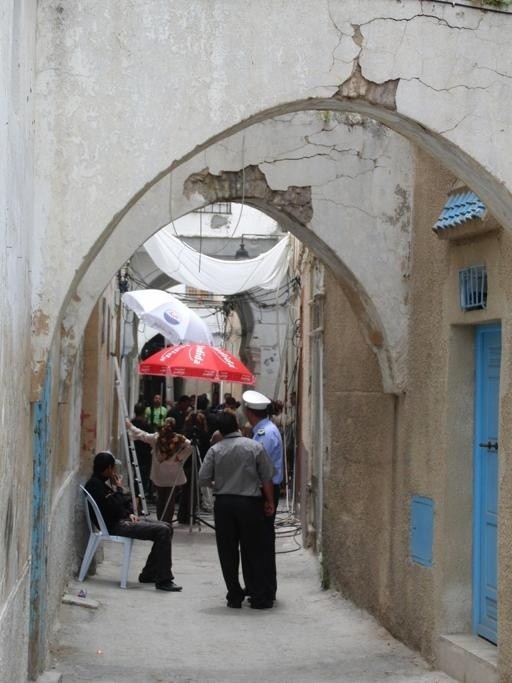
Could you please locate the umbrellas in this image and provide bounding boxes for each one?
[137,344,256,387]
[121,287,213,344]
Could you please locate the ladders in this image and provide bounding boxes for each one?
[116,381,150,516]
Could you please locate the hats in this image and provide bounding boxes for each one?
[241,389,271,410]
[93,452,122,469]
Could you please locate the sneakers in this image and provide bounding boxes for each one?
[139,572,183,592]
[225,587,274,610]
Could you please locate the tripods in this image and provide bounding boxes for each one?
[157,441,215,534]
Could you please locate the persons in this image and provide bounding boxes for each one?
[198,410,276,610]
[129,392,298,524]
[82,449,183,590]
[240,389,284,602]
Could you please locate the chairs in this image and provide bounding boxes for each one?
[77,484,134,589]
